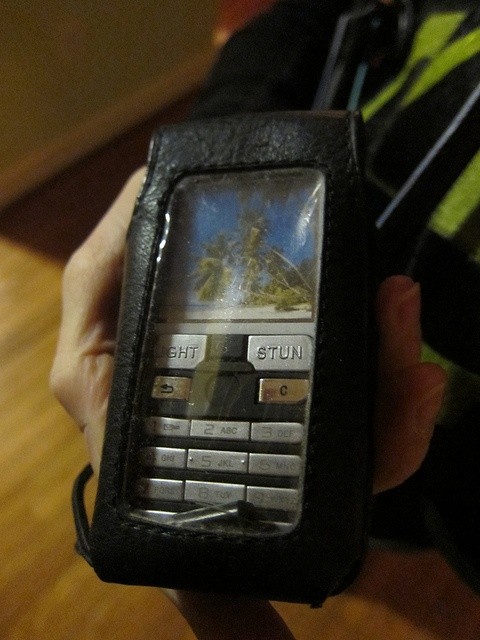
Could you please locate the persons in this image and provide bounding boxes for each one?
[49,0,480,640]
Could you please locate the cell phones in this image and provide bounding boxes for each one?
[88,109,378,602]
